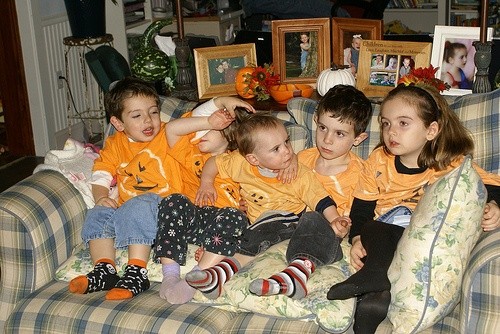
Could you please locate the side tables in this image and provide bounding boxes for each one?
[62,33,115,143]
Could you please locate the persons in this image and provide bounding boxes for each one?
[185,84,372,300]
[400,58,411,79]
[371,53,383,67]
[300,33,311,72]
[386,56,398,70]
[68,80,257,301]
[346,34,364,76]
[151,107,249,305]
[441,41,468,89]
[195,112,353,227]
[211,60,237,84]
[325,82,500,334]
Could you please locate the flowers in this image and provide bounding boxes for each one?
[397,64,450,98]
[242,65,283,100]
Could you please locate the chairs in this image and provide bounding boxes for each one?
[85,44,131,92]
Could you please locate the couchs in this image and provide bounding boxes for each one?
[0,96,500,334]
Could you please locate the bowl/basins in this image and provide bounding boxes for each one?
[271,84,312,104]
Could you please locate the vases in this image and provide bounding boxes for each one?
[64,0,106,38]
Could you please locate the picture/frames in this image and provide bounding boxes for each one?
[193,42,257,100]
[271,18,331,85]
[356,38,432,97]
[332,16,384,69]
[429,24,494,96]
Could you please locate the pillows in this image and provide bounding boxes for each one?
[51,153,490,334]
